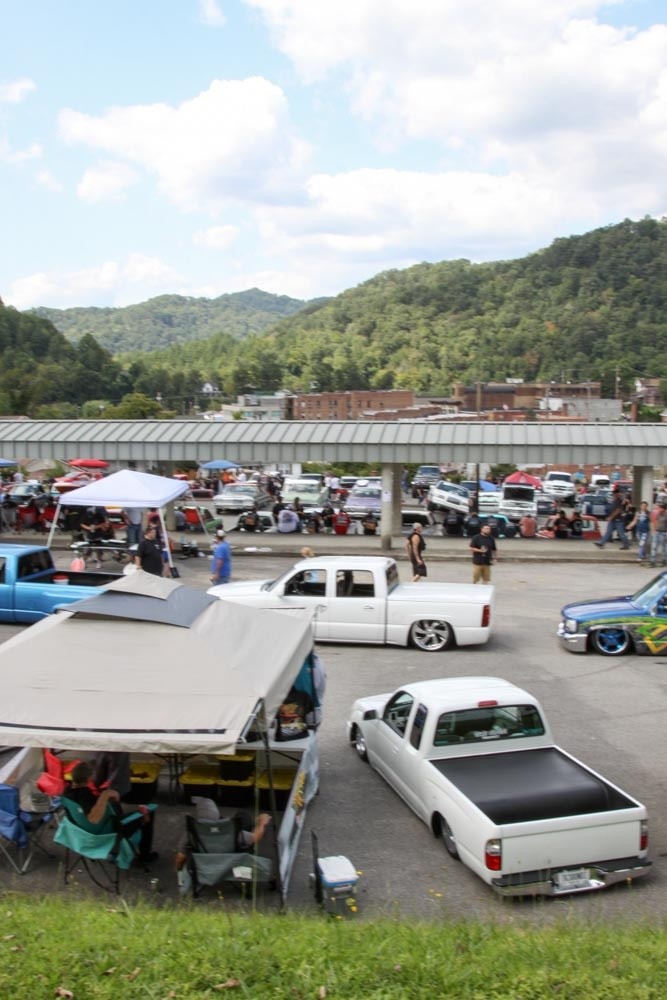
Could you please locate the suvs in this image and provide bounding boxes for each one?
[543,470,578,508]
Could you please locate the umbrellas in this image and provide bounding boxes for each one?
[201,460,240,468]
[0,458,109,467]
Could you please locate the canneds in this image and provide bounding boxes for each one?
[138,805,149,822]
[150,878,159,892]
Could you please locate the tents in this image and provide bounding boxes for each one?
[0,569,317,910]
[47,469,212,567]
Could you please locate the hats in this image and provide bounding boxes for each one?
[71,759,98,784]
[196,797,219,821]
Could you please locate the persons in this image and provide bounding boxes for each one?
[398,464,667,568]
[20,752,158,869]
[0,466,379,585]
[468,524,498,584]
[407,522,427,582]
[175,798,272,871]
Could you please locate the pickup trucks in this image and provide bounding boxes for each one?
[0,542,128,626]
[226,510,278,534]
[498,482,538,522]
[345,675,653,899]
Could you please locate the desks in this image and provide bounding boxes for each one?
[236,727,316,755]
[70,539,161,572]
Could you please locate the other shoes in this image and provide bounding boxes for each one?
[132,852,159,868]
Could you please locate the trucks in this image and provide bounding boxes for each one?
[202,555,497,653]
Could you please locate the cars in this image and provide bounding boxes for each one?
[533,491,558,515]
[408,463,539,537]
[400,510,443,538]
[580,472,635,519]
[278,470,385,533]
[557,571,667,654]
[0,480,62,529]
[170,505,223,531]
[213,480,272,513]
[54,471,104,485]
[538,513,604,541]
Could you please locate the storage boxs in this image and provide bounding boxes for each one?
[180,765,216,801]
[126,763,159,804]
[217,754,254,782]
[317,855,356,898]
[256,767,293,809]
[217,778,255,805]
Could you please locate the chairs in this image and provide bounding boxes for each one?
[178,816,276,903]
[335,581,344,596]
[47,790,158,899]
[0,781,60,877]
[346,573,353,593]
